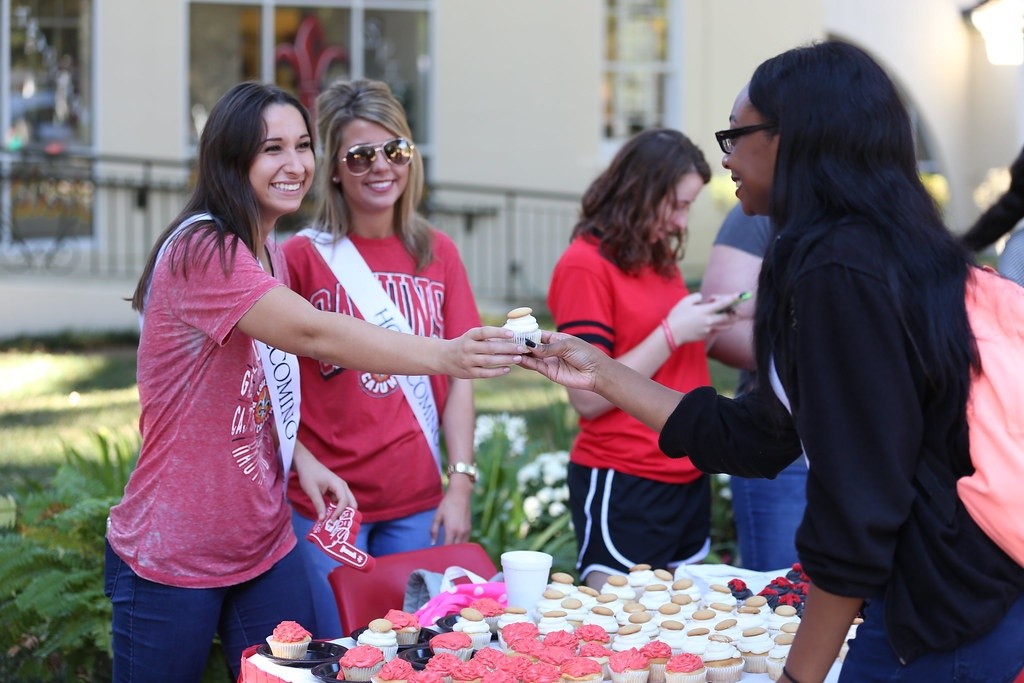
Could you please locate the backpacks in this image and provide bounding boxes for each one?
[917,264,1023,572]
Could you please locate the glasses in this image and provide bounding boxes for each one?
[340,136,414,175]
[714,122,778,155]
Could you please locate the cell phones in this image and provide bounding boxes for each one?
[716,291,752,314]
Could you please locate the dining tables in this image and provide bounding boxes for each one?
[242,565,877,683]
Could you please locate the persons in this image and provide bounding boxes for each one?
[547,127,735,594]
[279,78,481,640]
[517,38,1024,683]
[700,202,808,572]
[107,82,523,683]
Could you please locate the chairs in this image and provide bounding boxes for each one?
[326,542,501,637]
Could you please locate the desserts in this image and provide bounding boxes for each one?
[266,563,863,683]
[500,307,542,353]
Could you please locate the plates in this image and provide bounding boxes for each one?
[255,613,497,683]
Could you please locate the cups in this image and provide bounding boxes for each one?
[501,550,552,609]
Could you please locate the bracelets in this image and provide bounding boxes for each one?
[783,667,798,683]
[447,462,479,482]
[661,319,677,352]
